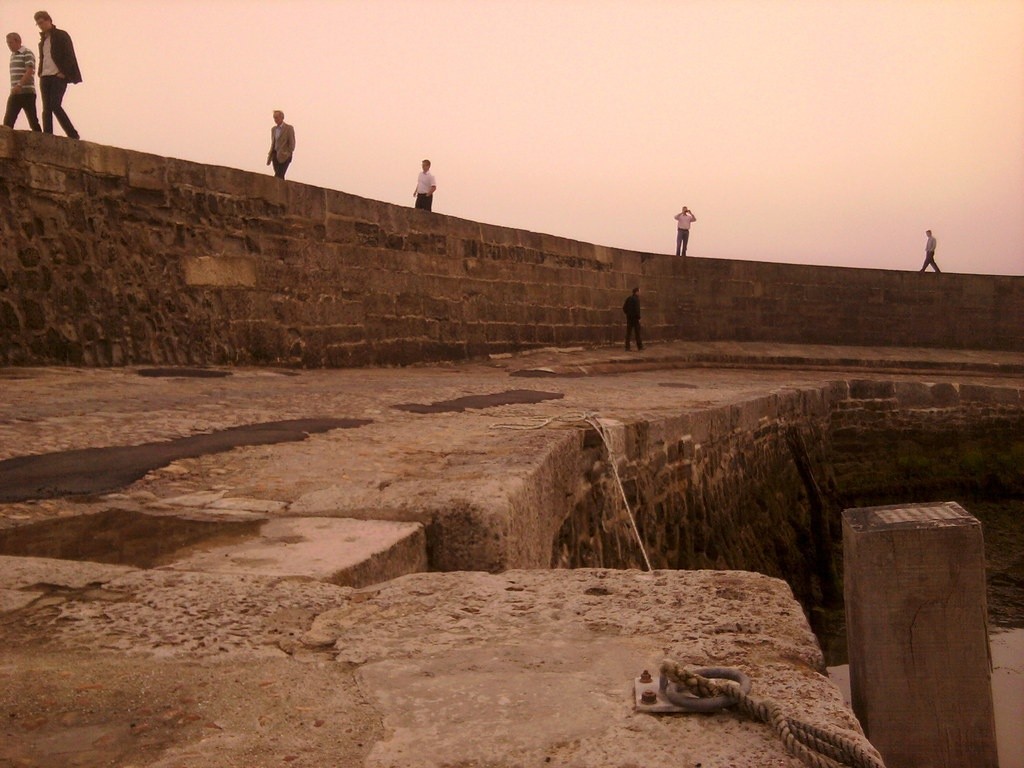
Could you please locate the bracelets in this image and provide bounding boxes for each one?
[17,83,22,87]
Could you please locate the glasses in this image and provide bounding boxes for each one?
[36,20,46,26]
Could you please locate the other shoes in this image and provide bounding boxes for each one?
[638,348,646,351]
[625,349,632,352]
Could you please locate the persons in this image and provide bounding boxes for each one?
[3,32,43,132]
[266,110,296,180]
[34,10,82,139]
[623,287,648,352]
[921,230,941,272]
[675,206,696,256]
[413,160,437,212]
